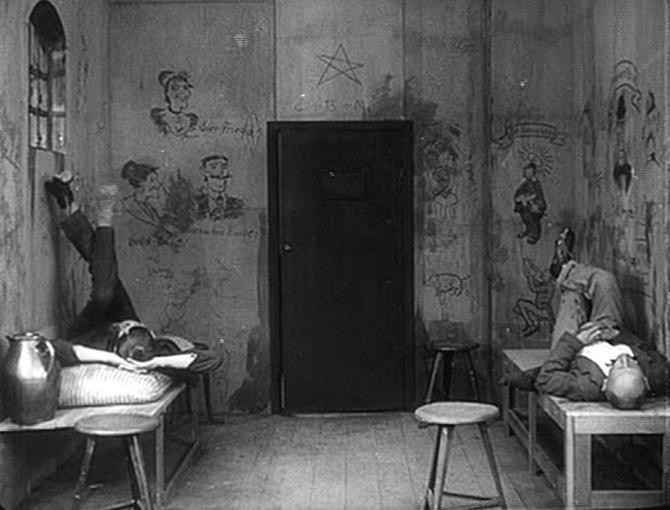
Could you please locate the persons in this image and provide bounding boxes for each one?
[643,134,662,264]
[192,154,246,221]
[613,95,632,194]
[114,162,186,257]
[514,162,548,242]
[43,171,224,375]
[149,69,199,138]
[535,227,670,410]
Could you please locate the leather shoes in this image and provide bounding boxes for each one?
[548,224,576,278]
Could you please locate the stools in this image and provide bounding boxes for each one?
[414,400,507,509]
[71,413,161,510]
[414,338,480,430]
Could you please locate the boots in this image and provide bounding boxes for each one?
[44,169,81,222]
[91,184,119,225]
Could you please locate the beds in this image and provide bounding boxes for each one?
[1,345,211,506]
[501,348,670,510]
